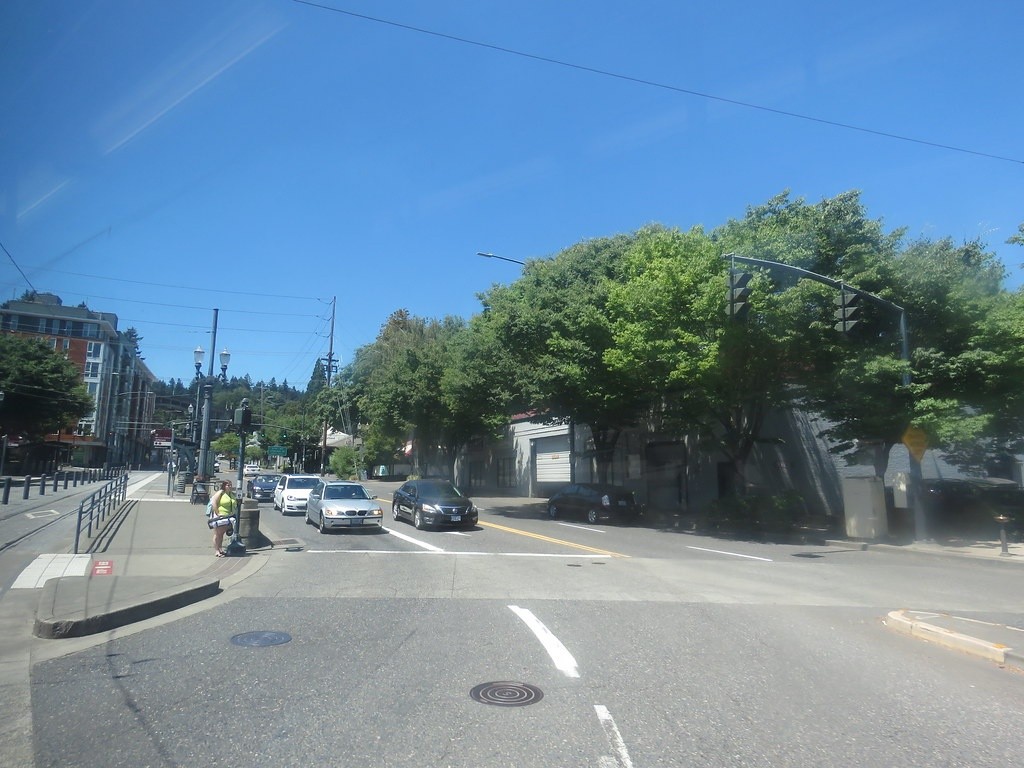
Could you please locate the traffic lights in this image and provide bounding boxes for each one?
[735,273,753,315]
[724,276,731,315]
[833,295,845,332]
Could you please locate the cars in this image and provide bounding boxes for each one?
[214,463,220,472]
[547,484,640,525]
[243,464,261,477]
[305,480,383,534]
[273,474,325,515]
[391,479,478,530]
[247,474,282,502]
[217,453,225,459]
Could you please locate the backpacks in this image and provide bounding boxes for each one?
[206,496,214,516]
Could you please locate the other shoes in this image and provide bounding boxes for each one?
[215,553,225,558]
[221,549,227,554]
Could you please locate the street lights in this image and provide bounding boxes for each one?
[845,294,862,331]
[56,373,120,463]
[190,346,230,504]
[188,404,204,442]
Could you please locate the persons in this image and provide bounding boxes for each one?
[209,480,238,556]
